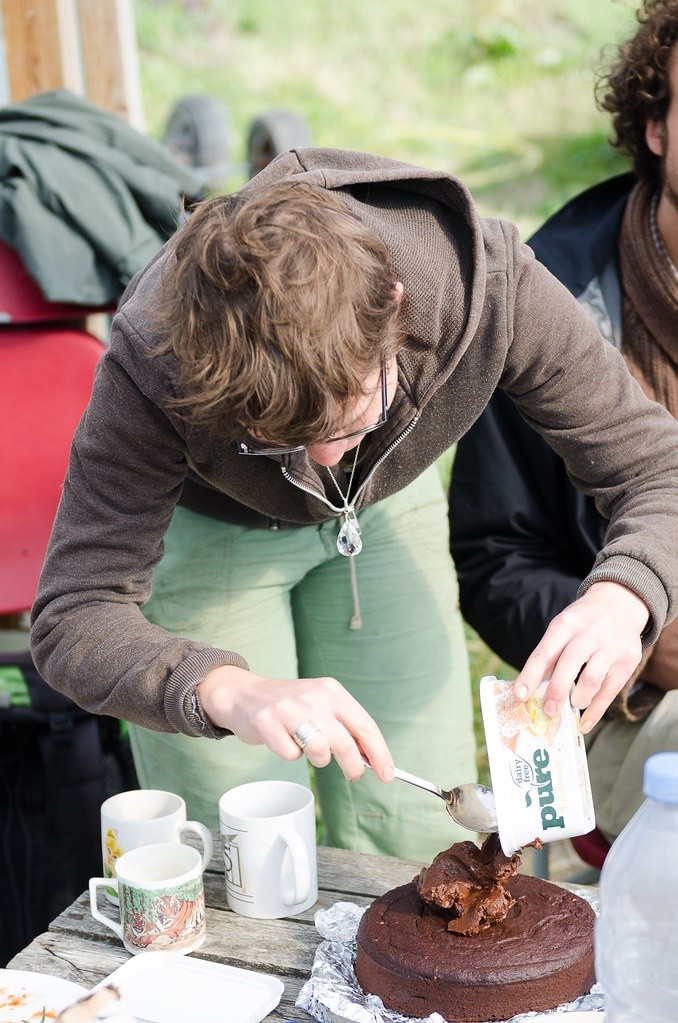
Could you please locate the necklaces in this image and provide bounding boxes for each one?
[320,439,366,557]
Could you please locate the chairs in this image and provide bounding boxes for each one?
[1,137,126,672]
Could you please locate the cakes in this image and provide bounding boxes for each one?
[354,829,597,1023]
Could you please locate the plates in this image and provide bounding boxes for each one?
[87,951,284,1023]
[0,967,89,1022]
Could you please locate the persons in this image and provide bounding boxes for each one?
[26,139,678,883]
[440,0,677,848]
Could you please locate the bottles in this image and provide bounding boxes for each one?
[596,752,678,1022]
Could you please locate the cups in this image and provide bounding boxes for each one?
[101,790,212,906]
[88,842,207,957]
[218,781,319,918]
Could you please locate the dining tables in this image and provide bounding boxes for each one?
[0,824,603,1021]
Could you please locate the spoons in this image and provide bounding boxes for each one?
[331,747,498,832]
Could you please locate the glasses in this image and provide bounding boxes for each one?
[224,348,397,460]
